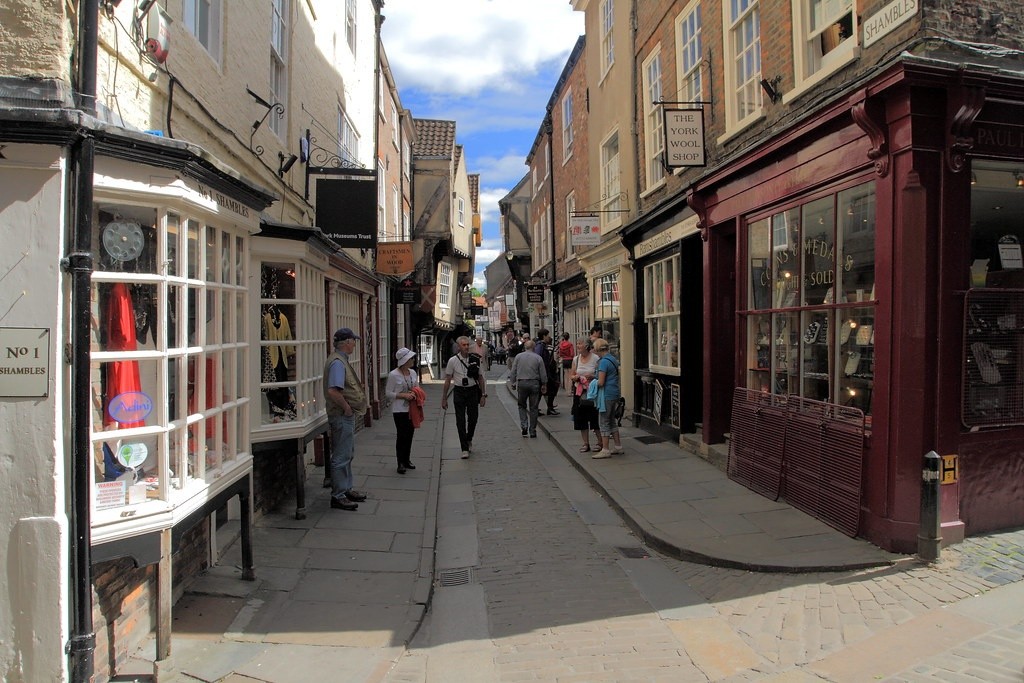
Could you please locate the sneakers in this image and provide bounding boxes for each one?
[330,496,358,510]
[344,490,366,502]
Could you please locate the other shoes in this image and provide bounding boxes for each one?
[571,415,574,421]
[404,460,415,469]
[553,405,558,408]
[522,430,528,437]
[592,448,611,458]
[397,465,406,473]
[610,446,624,454]
[530,431,536,438]
[538,412,545,416]
[467,434,472,452]
[547,409,560,415]
[461,451,469,459]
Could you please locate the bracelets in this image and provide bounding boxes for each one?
[482,394,485,396]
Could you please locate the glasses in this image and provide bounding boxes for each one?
[410,357,413,360]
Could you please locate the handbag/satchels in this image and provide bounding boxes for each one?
[614,397,625,427]
[478,388,483,404]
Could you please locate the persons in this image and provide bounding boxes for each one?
[510,341,548,438]
[589,327,601,353]
[386,348,419,473]
[507,329,560,415]
[451,338,458,355]
[592,339,623,458]
[469,335,507,398]
[442,336,485,458]
[560,332,575,396]
[569,336,603,452]
[323,328,366,510]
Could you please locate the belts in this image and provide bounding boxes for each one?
[519,379,538,381]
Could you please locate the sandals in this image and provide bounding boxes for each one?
[592,444,602,452]
[579,443,590,452]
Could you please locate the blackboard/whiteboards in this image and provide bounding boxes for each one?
[653,378,663,425]
[669,383,681,429]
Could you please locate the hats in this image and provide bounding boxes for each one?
[396,348,416,367]
[334,328,360,342]
